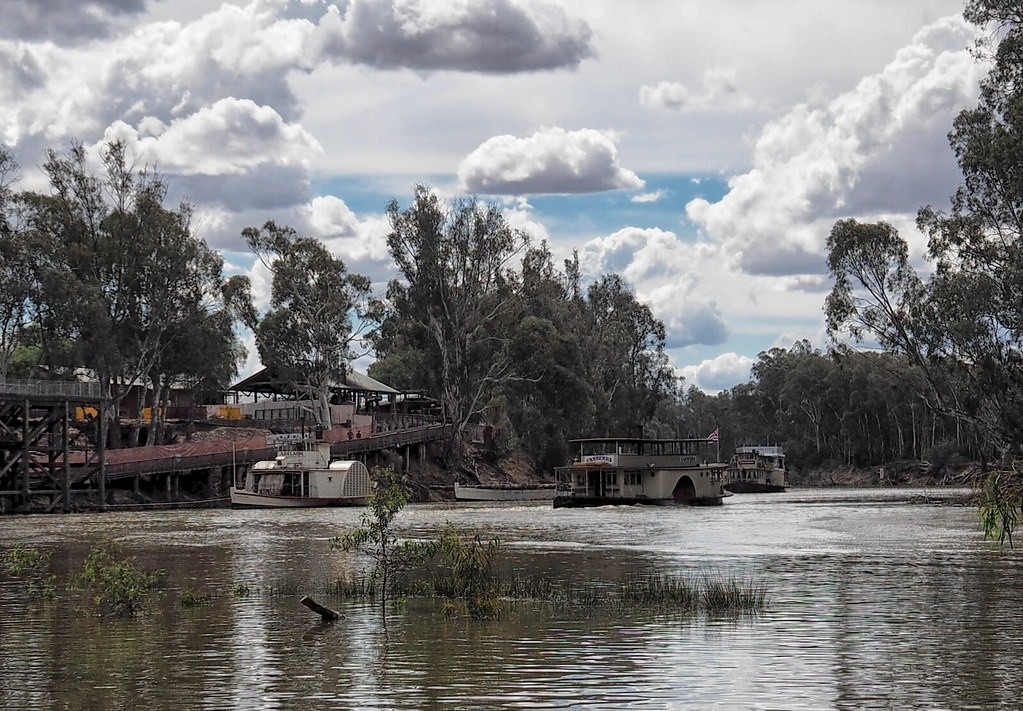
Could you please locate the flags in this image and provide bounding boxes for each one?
[706,427,718,445]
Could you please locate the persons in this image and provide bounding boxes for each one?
[357,431,361,439]
[347,430,353,440]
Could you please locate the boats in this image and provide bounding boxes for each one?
[552,436,734,509]
[228,438,376,508]
[721,446,788,494]
